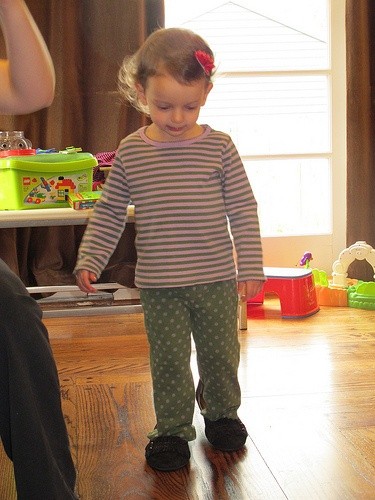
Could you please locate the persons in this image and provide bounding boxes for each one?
[0,0,77,500]
[73,27,267,472]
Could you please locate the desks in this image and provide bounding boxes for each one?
[0,206,247,330]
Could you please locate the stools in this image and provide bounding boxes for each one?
[252,267,320,319]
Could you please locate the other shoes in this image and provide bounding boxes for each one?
[145,435,190,471]
[203,415,248,450]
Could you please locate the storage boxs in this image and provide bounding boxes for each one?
[0,151,99,210]
[68,191,101,210]
